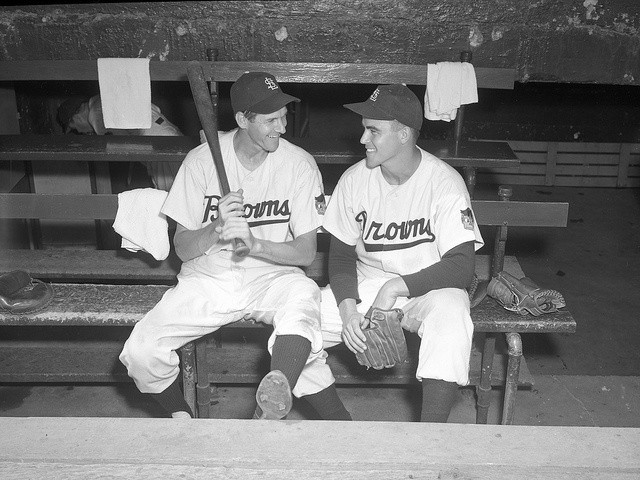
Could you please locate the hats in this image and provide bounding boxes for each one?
[230,70,300,114]
[343,82,423,131]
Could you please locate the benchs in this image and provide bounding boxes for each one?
[0,49,579,425]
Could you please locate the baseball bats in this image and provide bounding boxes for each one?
[186,60,251,257]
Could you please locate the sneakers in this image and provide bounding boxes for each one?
[251,370,293,419]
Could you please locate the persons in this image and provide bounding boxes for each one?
[119,72,325,418]
[56,99,191,190]
[268,82,485,423]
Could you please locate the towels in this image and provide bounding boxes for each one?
[97,57,152,130]
[112,187,170,262]
[423,61,479,123]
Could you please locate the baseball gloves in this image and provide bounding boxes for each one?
[485,270,567,316]
[355,306,409,371]
